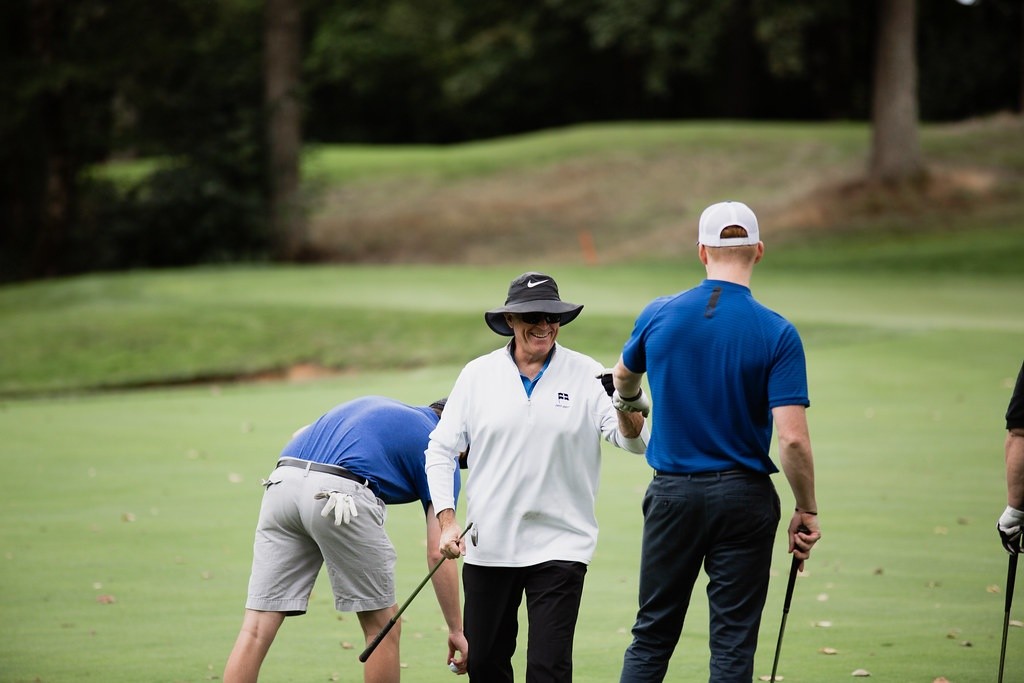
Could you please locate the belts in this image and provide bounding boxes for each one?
[277,459,375,490]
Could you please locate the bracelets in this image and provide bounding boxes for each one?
[795,508,818,515]
[619,388,642,402]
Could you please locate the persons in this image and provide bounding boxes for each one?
[611,201,821,683]
[222,398,468,683]
[996,361,1024,555]
[423,271,650,683]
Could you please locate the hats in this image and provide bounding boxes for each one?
[431,398,468,470]
[697,200,760,247]
[484,273,583,336]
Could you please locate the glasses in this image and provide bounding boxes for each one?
[513,311,561,325]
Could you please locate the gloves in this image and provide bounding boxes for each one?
[595,367,616,396]
[996,505,1024,554]
[612,388,650,419]
[314,490,358,526]
